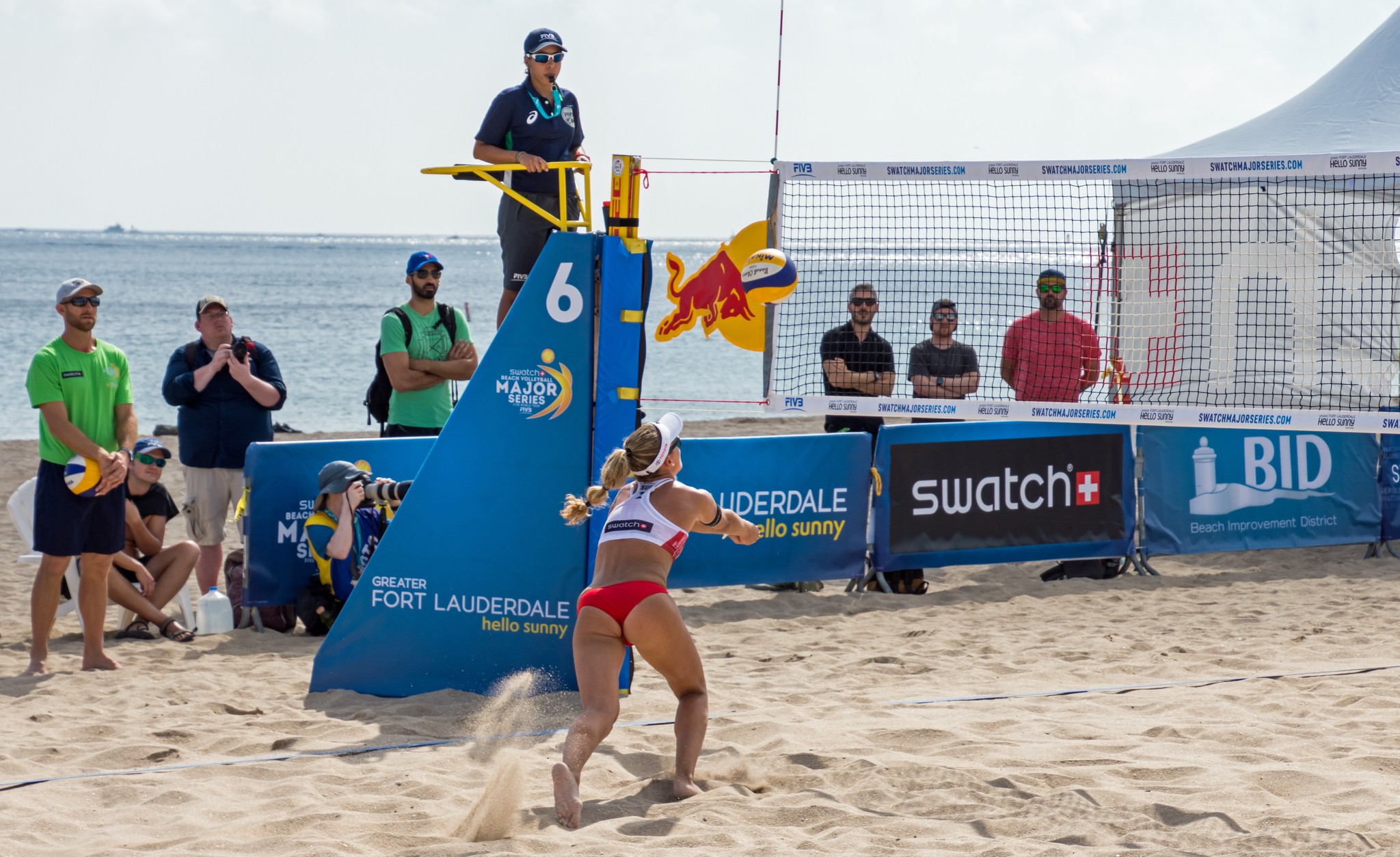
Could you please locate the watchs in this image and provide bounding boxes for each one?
[872,369,880,383]
[937,375,944,386]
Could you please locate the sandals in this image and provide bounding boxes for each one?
[160,617,195,642]
[114,619,155,640]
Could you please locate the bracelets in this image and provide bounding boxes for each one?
[515,150,526,162]
[573,153,591,161]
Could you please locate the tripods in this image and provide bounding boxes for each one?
[232,336,253,364]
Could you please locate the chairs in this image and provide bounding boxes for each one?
[6,476,195,630]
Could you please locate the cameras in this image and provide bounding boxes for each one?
[355,477,414,508]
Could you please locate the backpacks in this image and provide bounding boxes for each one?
[1040,558,1120,582]
[866,552,925,594]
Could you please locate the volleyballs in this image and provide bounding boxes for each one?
[64,454,102,498]
[741,248,798,307]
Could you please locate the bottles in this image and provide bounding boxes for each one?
[196,586,234,635]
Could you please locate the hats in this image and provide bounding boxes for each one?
[524,28,568,53]
[56,278,103,304]
[195,295,229,315]
[630,412,682,477]
[1037,269,1066,286]
[929,299,957,332]
[133,437,172,459]
[406,251,444,275]
[313,460,373,513]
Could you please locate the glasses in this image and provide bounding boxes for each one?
[133,454,166,467]
[849,297,877,307]
[932,312,958,322]
[60,297,100,307]
[409,270,441,279]
[202,310,228,320]
[524,53,565,63]
[1037,285,1064,293]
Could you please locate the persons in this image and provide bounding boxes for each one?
[907,298,977,425]
[110,438,199,641]
[473,25,586,330]
[820,284,896,463]
[552,408,760,830]
[999,268,1101,417]
[161,297,288,630]
[365,252,481,436]
[302,461,414,615]
[24,278,136,676]
